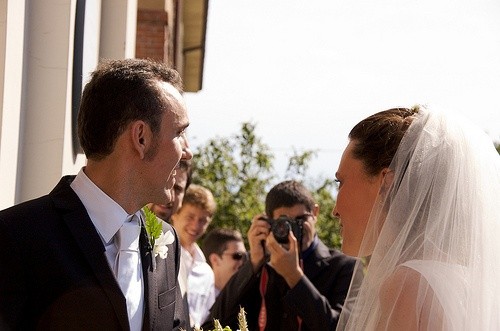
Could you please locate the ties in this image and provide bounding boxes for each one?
[113,217,144,330]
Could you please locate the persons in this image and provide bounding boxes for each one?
[202,226,248,301]
[0,56,194,331]
[332,99,500,331]
[198,181,365,331]
[168,183,216,329]
[145,160,195,224]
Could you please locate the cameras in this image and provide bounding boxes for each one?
[264,215,303,244]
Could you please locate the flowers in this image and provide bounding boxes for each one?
[142,204,175,260]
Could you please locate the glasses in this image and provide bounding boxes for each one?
[222,252,242,260]
